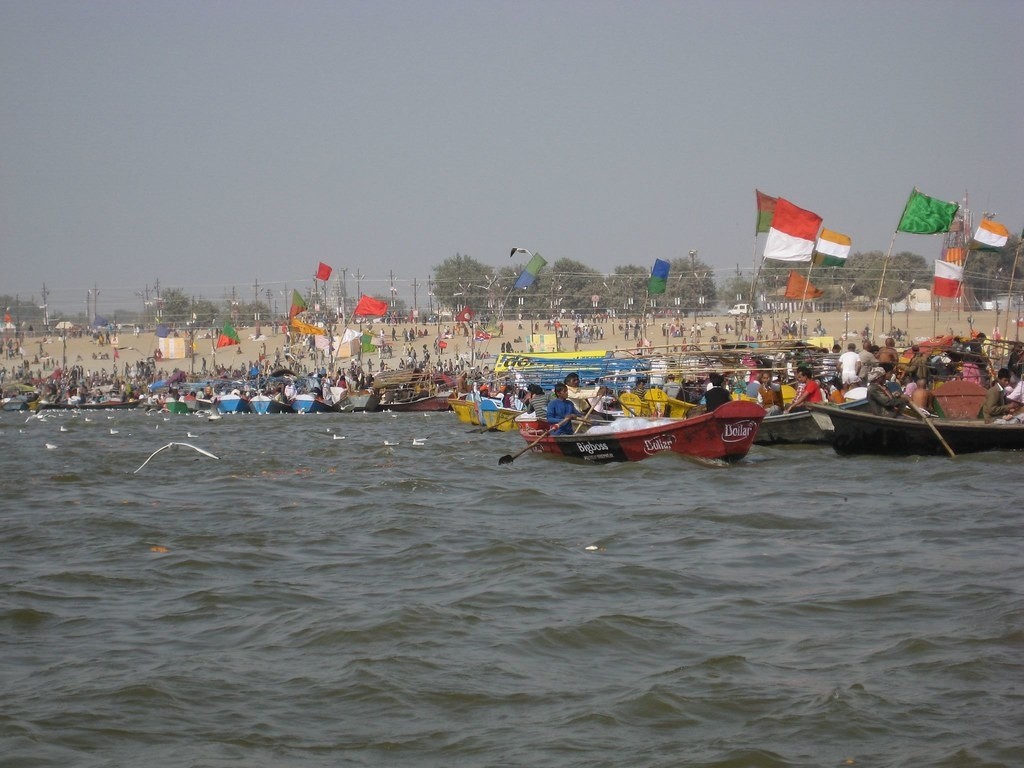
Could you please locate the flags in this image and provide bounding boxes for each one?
[354,294,388,317]
[755,187,778,236]
[316,261,332,280]
[290,317,326,335]
[933,258,963,299]
[214,321,241,348]
[895,186,960,234]
[940,246,965,262]
[786,270,824,299]
[646,257,670,294]
[967,216,1010,254]
[287,288,308,319]
[761,196,823,262]
[513,252,548,288]
[813,226,851,266]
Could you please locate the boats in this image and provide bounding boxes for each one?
[1,335,1023,465]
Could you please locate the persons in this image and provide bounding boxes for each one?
[1,304,1024,442]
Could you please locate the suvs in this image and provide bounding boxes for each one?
[728,304,753,318]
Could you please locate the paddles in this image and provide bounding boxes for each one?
[464,385,640,466]
[906,395,957,459]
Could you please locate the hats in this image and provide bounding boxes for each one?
[868,367,886,383]
